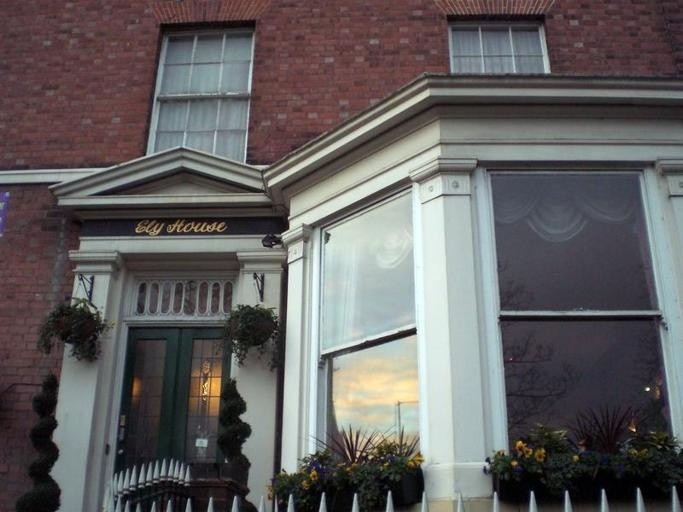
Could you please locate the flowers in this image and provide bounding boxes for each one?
[263,424,424,509]
[484,404,682,488]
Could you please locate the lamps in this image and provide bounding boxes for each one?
[259,233,282,248]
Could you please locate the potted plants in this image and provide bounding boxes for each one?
[31,296,125,362]
[214,303,285,371]
